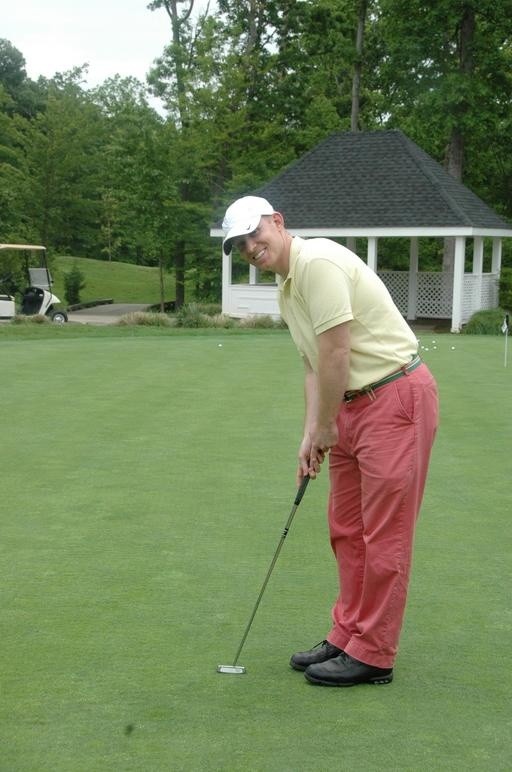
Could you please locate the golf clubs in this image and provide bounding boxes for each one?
[218,475,308,675]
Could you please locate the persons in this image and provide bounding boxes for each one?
[222,194,437,688]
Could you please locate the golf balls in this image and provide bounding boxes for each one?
[218,344,222,347]
[418,338,455,352]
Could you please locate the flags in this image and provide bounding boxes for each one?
[500,314,510,333]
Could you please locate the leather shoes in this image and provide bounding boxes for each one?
[305,650,393,687]
[289,640,345,671]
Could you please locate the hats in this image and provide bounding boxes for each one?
[220,194,276,256]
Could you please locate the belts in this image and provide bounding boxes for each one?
[343,350,422,405]
